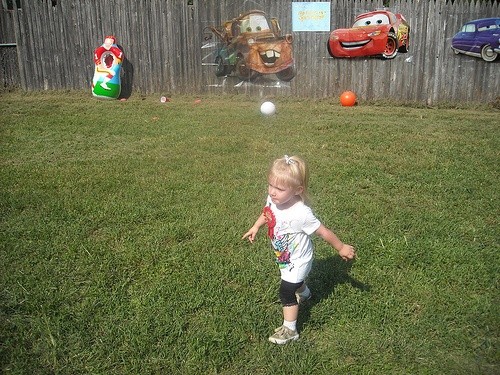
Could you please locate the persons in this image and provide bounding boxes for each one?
[241,154,355,345]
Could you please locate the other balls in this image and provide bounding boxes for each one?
[261,102,276,116]
[340,91,356,106]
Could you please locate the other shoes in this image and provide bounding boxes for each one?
[269,325,299,344]
[296,284,311,303]
